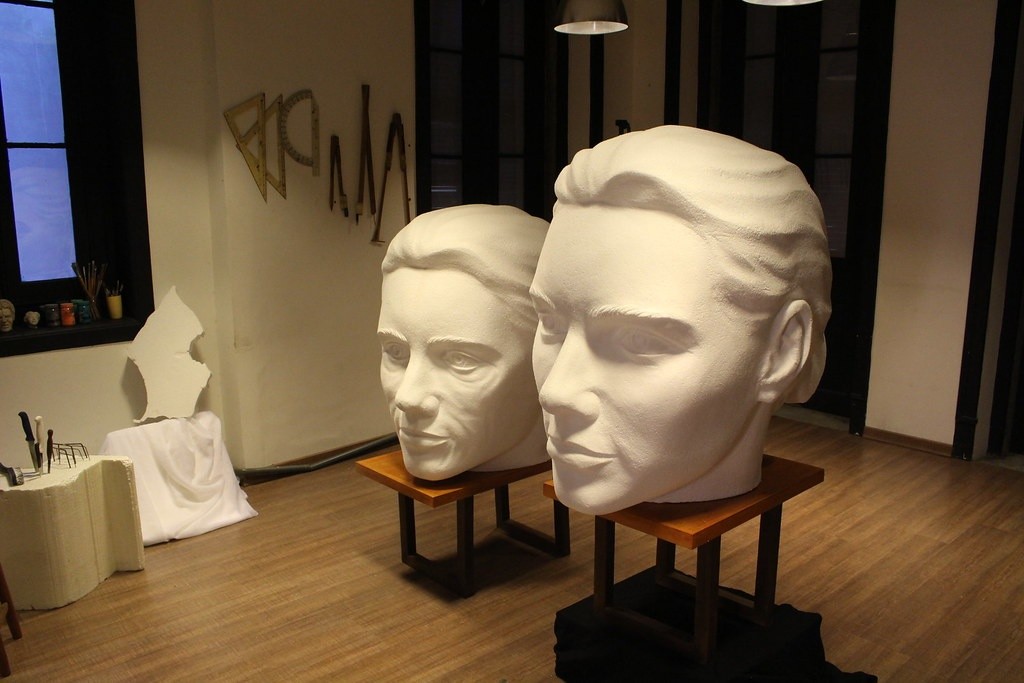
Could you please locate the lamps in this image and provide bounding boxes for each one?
[552,0,628,34]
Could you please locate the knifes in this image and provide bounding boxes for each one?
[18,411,53,479]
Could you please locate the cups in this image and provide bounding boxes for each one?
[59,303,76,326]
[77,301,92,323]
[71,298,82,320]
[45,303,61,328]
[107,294,122,320]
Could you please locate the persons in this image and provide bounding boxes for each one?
[378,204,550,478]
[24,311,40,330]
[0,299,15,332]
[529,126,832,515]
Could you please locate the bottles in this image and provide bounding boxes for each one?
[87,296,103,321]
